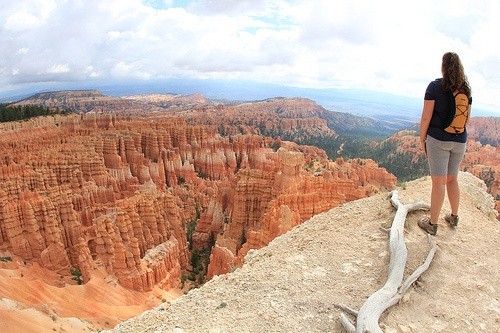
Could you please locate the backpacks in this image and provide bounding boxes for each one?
[440,87,472,134]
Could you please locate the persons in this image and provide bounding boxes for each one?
[417,51,474,235]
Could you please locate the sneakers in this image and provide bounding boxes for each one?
[446,213,459,227]
[417,217,438,235]
